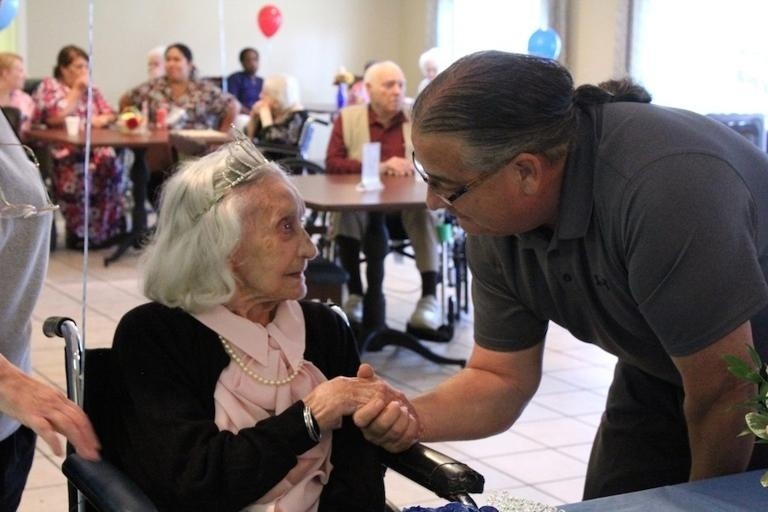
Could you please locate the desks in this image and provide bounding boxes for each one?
[288,174,466,369]
[24,129,168,267]
[555,470,768,512]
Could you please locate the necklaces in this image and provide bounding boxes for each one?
[219,336,304,386]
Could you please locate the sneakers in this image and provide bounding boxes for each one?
[411,294,445,331]
[341,293,364,322]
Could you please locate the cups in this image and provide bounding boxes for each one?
[65,116,79,136]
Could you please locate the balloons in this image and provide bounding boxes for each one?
[528,28,562,60]
[258,4,281,39]
[1,1,19,30]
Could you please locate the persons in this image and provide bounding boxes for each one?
[1,112,98,511]
[353,51,768,505]
[1,45,312,254]
[324,47,452,331]
[96,122,422,511]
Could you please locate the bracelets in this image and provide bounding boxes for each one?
[303,408,323,442]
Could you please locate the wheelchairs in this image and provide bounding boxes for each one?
[250,108,329,172]
[304,173,470,354]
[40,314,486,512]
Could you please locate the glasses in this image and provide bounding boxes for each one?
[0,143,61,220]
[411,150,543,205]
